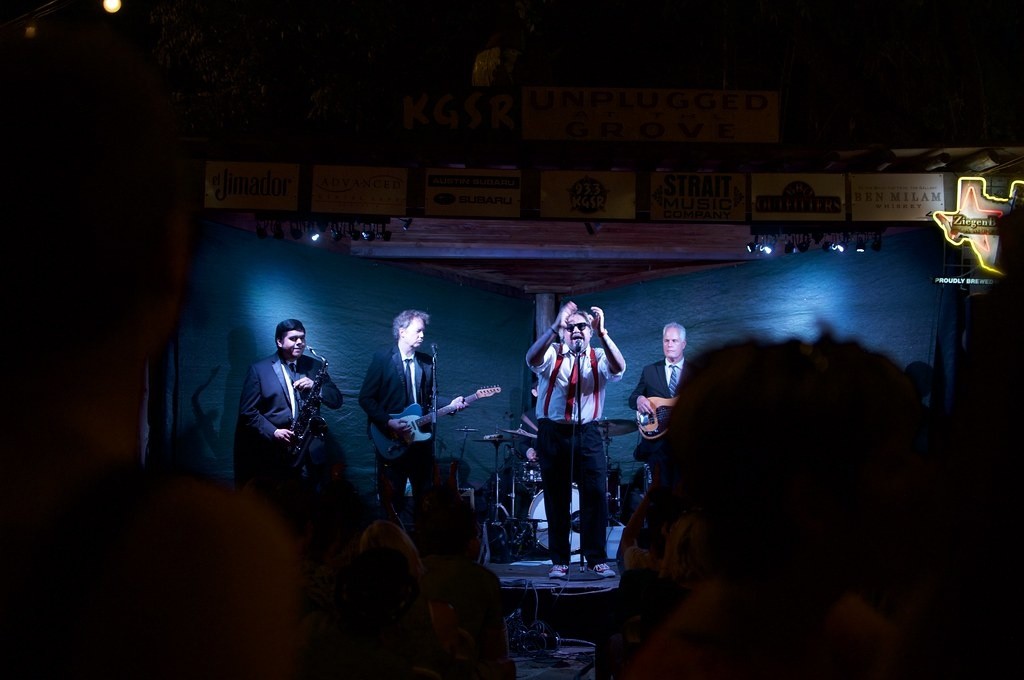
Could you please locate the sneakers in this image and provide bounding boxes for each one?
[587,563,616,578]
[547,563,569,578]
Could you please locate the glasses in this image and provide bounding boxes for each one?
[564,321,591,332]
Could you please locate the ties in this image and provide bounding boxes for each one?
[289,363,297,380]
[404,359,415,407]
[668,366,678,398]
[565,350,585,423]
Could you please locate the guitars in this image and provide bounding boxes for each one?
[636,396,678,440]
[371,385,502,464]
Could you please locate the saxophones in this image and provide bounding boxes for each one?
[284,349,327,471]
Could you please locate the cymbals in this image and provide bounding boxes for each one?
[499,429,537,440]
[473,439,525,445]
[598,418,638,437]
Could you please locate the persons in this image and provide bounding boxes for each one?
[0,19,318,680]
[609,343,961,679]
[240,319,343,542]
[627,322,700,466]
[358,308,438,555]
[524,301,626,579]
[512,408,540,466]
[237,462,717,680]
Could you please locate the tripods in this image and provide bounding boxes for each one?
[487,443,509,525]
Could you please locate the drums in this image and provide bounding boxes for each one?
[529,483,601,555]
[606,469,622,514]
[512,460,543,481]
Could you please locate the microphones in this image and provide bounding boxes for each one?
[506,444,523,460]
[575,339,583,352]
[431,343,439,360]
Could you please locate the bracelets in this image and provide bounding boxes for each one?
[549,326,559,335]
